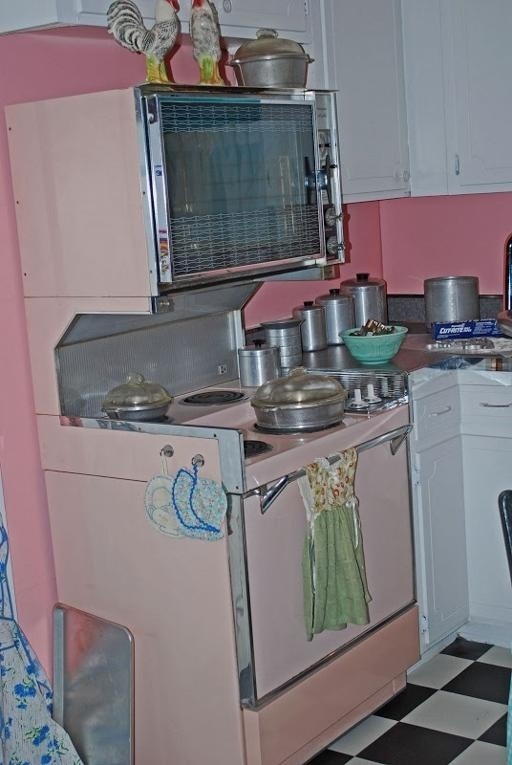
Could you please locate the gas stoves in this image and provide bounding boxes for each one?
[93,386,407,492]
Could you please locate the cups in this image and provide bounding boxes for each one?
[421,275,480,333]
[260,318,305,368]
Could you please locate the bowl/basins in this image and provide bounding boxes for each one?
[339,326,409,365]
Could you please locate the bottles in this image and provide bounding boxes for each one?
[292,271,387,352]
[238,339,282,387]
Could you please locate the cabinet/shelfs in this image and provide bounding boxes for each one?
[406,372,469,654]
[2,0,311,52]
[401,1,511,197]
[457,369,512,630]
[300,2,409,206]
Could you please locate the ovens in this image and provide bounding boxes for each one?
[240,427,417,704]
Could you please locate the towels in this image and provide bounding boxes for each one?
[300,494,373,642]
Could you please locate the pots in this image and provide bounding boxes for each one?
[228,27,314,89]
[101,373,172,421]
[250,368,345,429]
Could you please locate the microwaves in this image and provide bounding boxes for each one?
[141,87,347,288]
